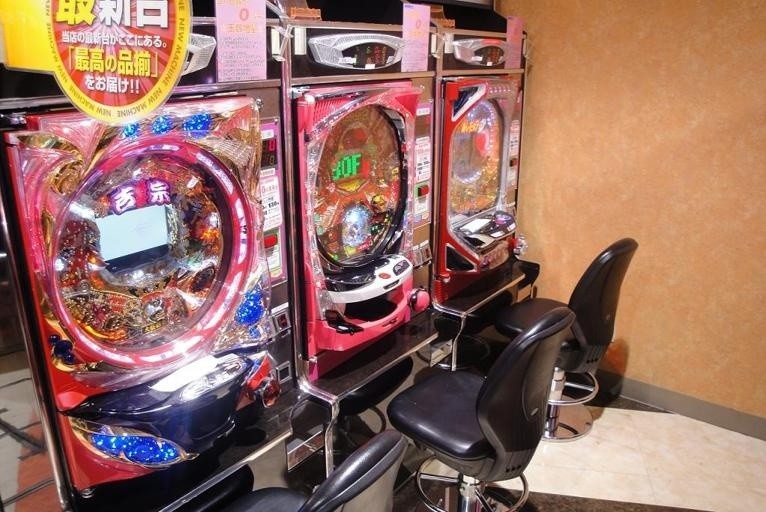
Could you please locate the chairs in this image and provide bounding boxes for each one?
[308,349,416,433]
[221,427,408,510]
[472,239,638,446]
[386,306,577,511]
[196,463,256,509]
